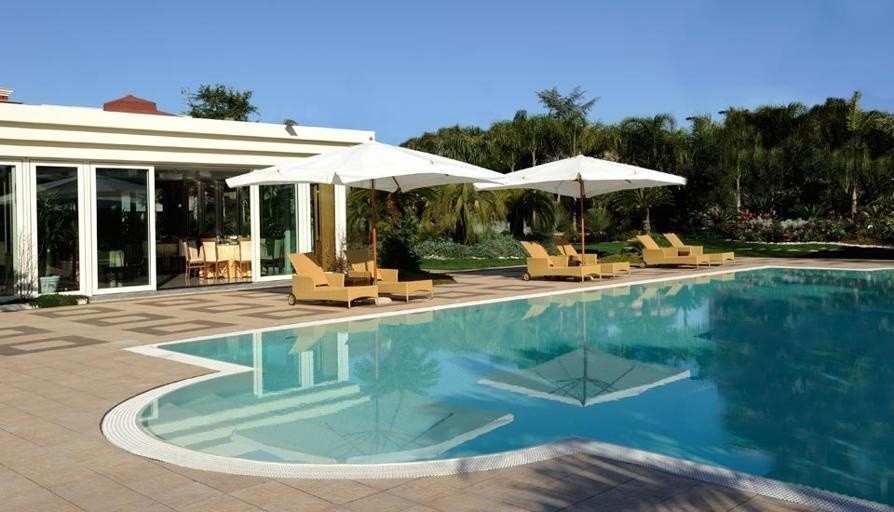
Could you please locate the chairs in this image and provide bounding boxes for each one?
[636,231,737,268]
[57,244,128,293]
[289,314,436,362]
[143,234,285,282]
[633,274,735,318]
[287,248,435,308]
[518,240,632,282]
[520,287,630,323]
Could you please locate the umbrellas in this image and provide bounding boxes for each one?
[225,135,509,281]
[474,148,687,258]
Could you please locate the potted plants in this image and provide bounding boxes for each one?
[37,189,72,296]
